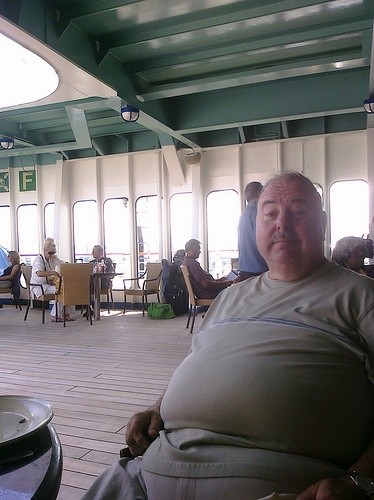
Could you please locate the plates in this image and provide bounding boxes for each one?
[0,395,53,443]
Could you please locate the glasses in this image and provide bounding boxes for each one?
[48,252,56,255]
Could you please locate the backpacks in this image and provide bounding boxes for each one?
[165,284,184,314]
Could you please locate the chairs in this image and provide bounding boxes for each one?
[54,262,94,329]
[0,262,27,311]
[122,261,164,318]
[180,264,215,335]
[160,259,199,317]
[21,264,63,325]
[80,263,117,315]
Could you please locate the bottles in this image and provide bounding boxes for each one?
[100,259,104,273]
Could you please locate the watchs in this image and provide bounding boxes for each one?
[347,469,374,500]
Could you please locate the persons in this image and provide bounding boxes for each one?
[182,239,233,299]
[82,245,115,317]
[0,251,20,308]
[81,170,374,500]
[236,181,268,283]
[29,238,75,323]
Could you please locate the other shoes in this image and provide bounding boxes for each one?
[83,310,93,317]
[51,316,64,322]
[0,304,3,307]
[62,314,75,321]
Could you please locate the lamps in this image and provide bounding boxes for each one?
[119,104,141,122]
[363,92,374,114]
[0,137,15,150]
[184,151,202,166]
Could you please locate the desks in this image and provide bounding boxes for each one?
[49,270,125,321]
[0,422,64,500]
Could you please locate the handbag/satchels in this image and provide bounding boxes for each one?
[148,302,175,319]
